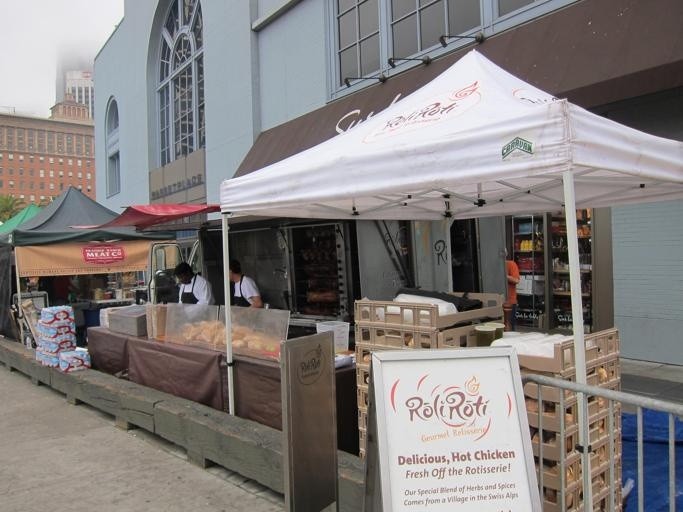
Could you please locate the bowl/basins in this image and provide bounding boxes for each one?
[103,292,113,299]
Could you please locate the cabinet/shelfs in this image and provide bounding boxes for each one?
[352,296,505,461]
[504,208,614,336]
[488,327,622,510]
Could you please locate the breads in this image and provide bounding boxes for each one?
[186,320,262,351]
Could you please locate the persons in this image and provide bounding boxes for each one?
[506,259,520,331]
[54,278,81,305]
[174,262,214,322]
[229,257,262,326]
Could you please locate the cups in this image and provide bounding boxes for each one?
[115,290,122,300]
[474,322,505,346]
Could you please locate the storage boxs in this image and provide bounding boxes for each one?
[32,305,89,372]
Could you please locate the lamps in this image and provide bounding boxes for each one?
[343,32,483,87]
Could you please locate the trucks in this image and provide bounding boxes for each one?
[146,215,508,338]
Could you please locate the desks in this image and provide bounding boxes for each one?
[85,325,354,430]
